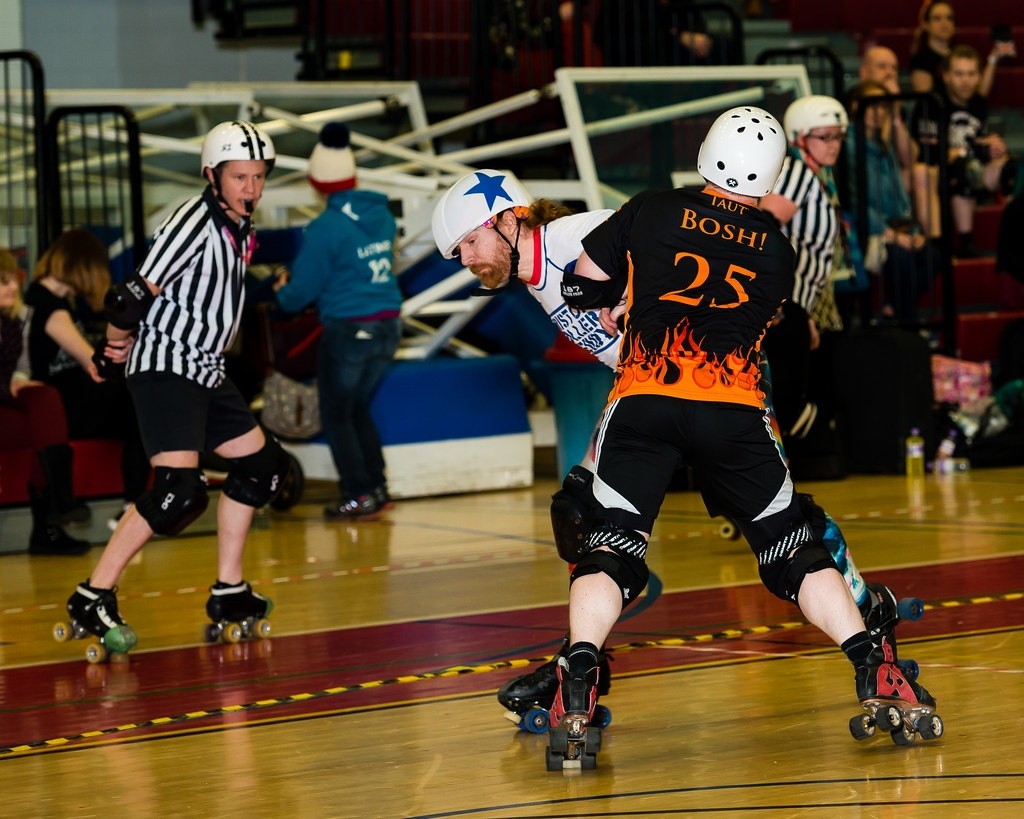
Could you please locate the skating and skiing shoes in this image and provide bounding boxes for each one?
[211,641,272,674]
[858,581,924,680]
[545,666,603,770]
[53,579,136,662]
[206,580,274,643]
[848,636,944,743]
[497,635,616,733]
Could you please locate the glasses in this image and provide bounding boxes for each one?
[805,132,844,141]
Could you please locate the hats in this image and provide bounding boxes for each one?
[306,121,357,192]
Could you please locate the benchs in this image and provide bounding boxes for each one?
[211,0,1023,381]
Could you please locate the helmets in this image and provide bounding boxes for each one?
[696,105,786,198]
[199,120,274,177]
[434,168,540,260]
[784,95,849,142]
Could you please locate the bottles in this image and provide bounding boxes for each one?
[906,430,924,478]
[934,432,956,477]
[927,459,968,473]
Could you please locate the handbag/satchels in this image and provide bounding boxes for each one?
[260,372,322,438]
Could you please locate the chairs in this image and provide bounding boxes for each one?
[0,377,126,505]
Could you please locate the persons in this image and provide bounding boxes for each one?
[843,41,919,341]
[272,119,402,520]
[0,227,151,557]
[593,0,718,171]
[432,168,899,714]
[907,0,1024,288]
[66,127,288,655]
[546,107,938,726]
[757,95,848,442]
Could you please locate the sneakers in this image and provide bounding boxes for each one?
[29,529,90,556]
[56,665,139,711]
[324,495,380,519]
[372,486,394,511]
[59,503,92,525]
